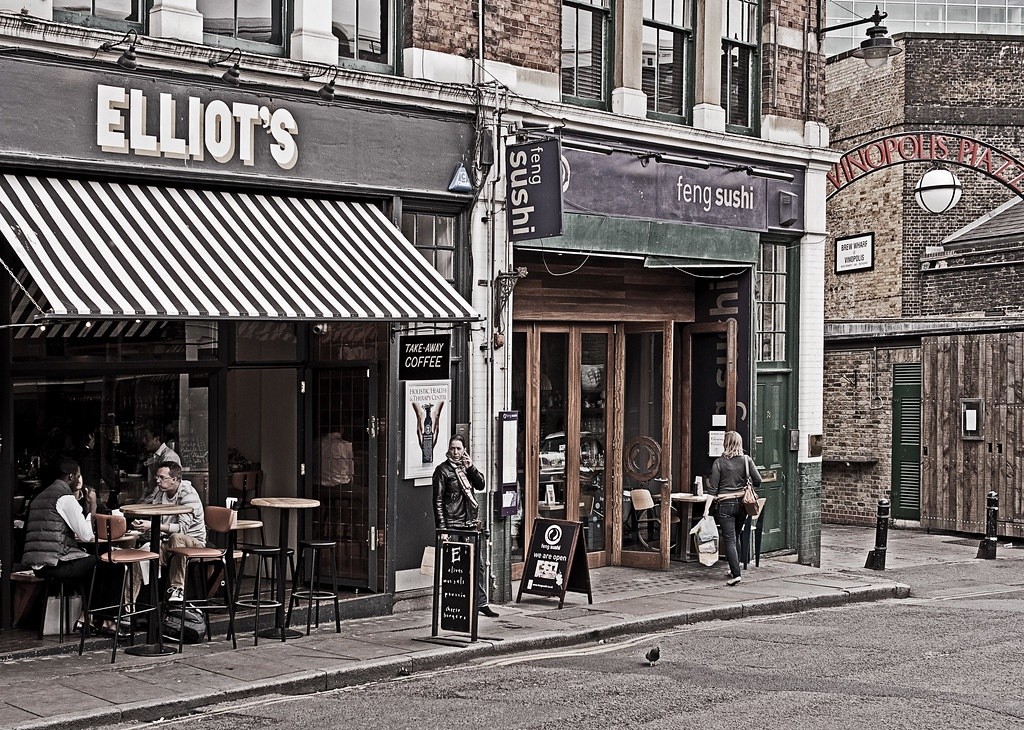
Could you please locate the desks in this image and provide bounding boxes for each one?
[75,530,140,637]
[651,493,716,562]
[538,501,584,519]
[205,520,263,613]
[251,498,320,640]
[119,504,194,657]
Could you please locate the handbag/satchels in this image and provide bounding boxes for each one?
[688,516,719,567]
[743,455,759,516]
[43,588,83,635]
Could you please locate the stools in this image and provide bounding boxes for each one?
[8,574,43,629]
[226,540,341,642]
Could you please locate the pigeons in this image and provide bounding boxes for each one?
[646,647,660,666]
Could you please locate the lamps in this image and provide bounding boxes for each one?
[303,65,337,102]
[101,28,138,71]
[914,161,962,215]
[208,47,242,86]
[820,5,902,67]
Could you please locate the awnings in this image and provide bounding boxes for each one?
[0,173,481,321]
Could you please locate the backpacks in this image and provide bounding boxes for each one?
[133,600,206,645]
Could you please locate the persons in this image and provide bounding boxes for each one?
[432,434,500,618]
[703,431,762,586]
[71,430,95,486]
[71,475,110,534]
[139,427,181,505]
[20,457,131,637]
[115,460,206,627]
[317,424,364,501]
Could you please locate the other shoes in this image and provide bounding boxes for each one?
[478,606,499,617]
[167,588,184,602]
[727,577,741,586]
[728,570,734,578]
[114,614,131,626]
[72,616,127,638]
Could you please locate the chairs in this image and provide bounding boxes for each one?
[717,498,767,571]
[162,506,238,654]
[630,489,680,552]
[579,495,595,517]
[78,513,164,664]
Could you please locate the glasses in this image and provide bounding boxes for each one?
[154,474,171,481]
